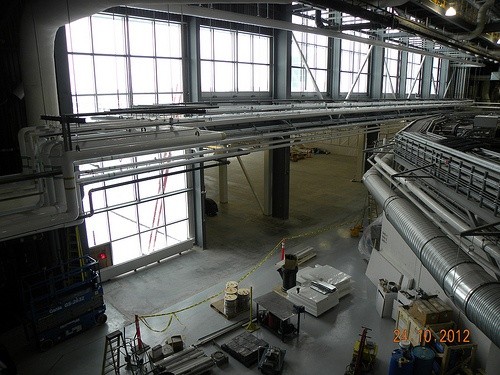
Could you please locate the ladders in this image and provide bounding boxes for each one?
[361,190,379,228]
[101,330,135,375]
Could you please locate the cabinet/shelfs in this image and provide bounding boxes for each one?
[377,284,397,318]
[391,290,413,321]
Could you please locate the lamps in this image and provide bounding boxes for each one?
[445,3,456,16]
